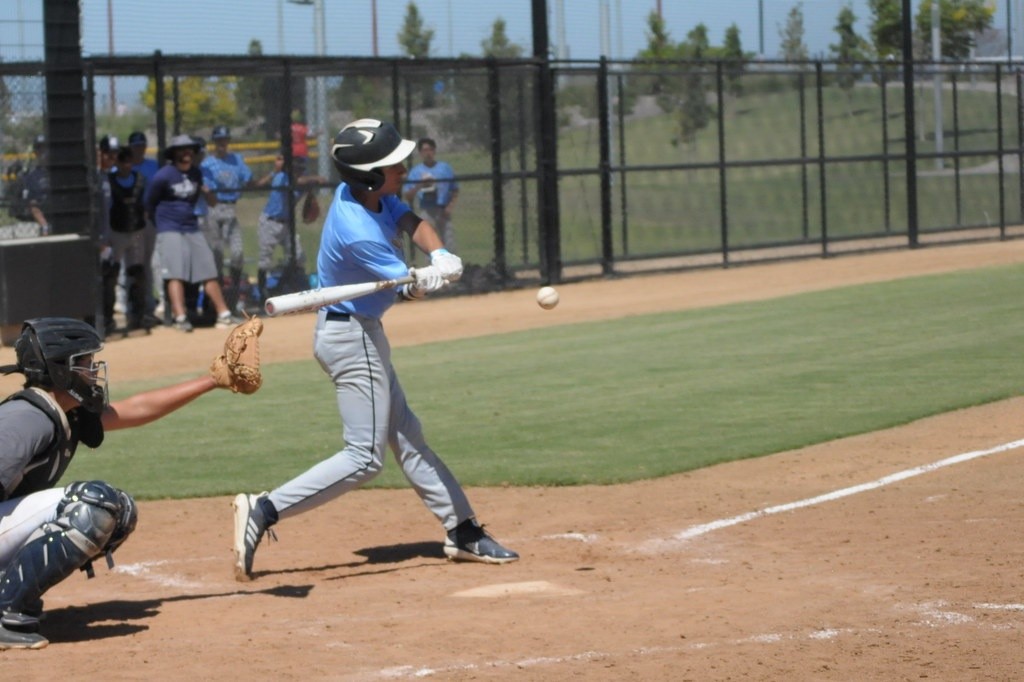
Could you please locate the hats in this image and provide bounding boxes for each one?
[212,125,231,140]
[162,135,202,159]
[130,133,147,147]
[100,135,119,152]
[34,135,47,152]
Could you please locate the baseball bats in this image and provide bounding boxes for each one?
[264,275,418,318]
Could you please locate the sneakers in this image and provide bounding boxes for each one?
[230,490,278,582]
[0,624,48,651]
[444,524,519,564]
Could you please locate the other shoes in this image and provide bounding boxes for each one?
[171,318,194,332]
[215,314,243,328]
[138,314,158,325]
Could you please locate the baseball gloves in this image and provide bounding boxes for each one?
[211,316,264,395]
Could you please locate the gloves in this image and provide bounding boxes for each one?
[408,266,446,298]
[430,250,464,281]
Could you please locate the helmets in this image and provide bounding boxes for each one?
[15,317,109,415]
[332,119,416,192]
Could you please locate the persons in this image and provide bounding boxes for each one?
[0,318,264,647]
[97,125,254,331]
[403,138,460,250]
[234,119,519,580]
[275,110,325,175]
[23,135,50,235]
[257,156,326,305]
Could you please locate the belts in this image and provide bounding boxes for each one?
[219,199,235,205]
[327,310,350,322]
[270,216,287,223]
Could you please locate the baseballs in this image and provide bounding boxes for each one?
[537,286,559,310]
